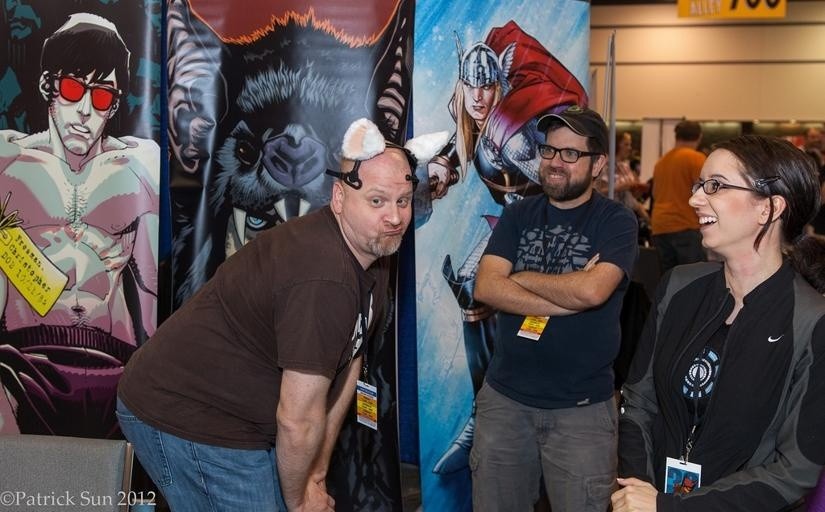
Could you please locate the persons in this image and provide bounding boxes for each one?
[609,134,825,511]
[469,109,641,510]
[593,111,825,299]
[114,115,415,512]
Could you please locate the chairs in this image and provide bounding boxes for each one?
[0,431,137,511]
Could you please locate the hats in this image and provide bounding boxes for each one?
[536,104,610,143]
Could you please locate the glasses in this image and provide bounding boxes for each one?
[532,143,603,162]
[50,71,122,112]
[686,177,761,196]
